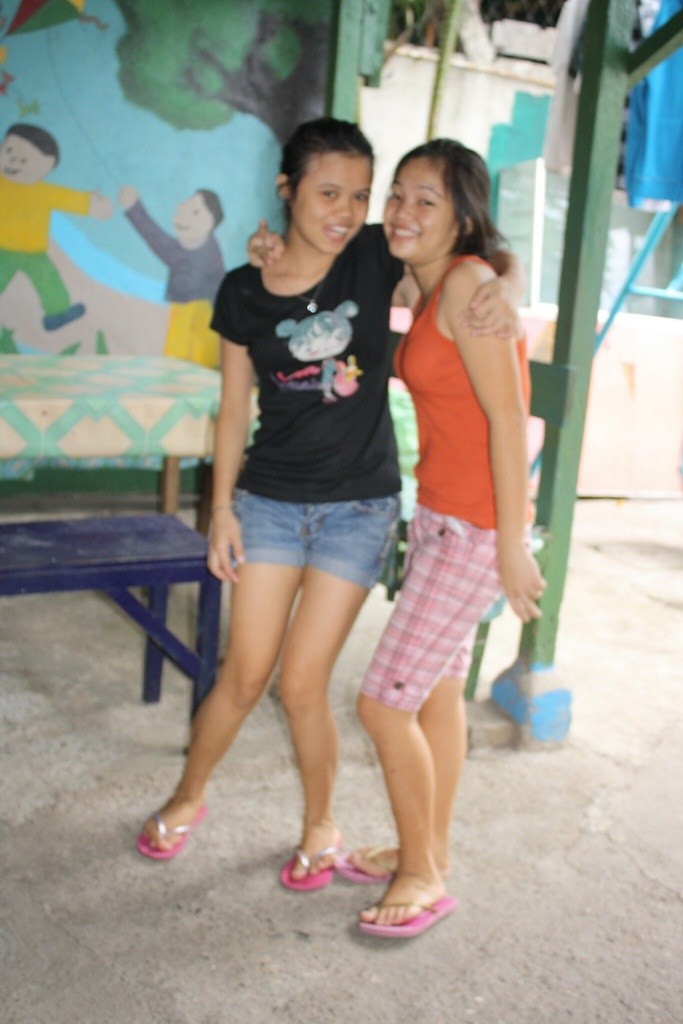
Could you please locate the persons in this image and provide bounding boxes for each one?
[246,137,548,939]
[135,117,527,891]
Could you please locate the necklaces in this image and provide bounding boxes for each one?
[297,279,325,313]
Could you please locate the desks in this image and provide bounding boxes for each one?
[0,354,261,598]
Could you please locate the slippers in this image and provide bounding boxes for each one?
[358,897,453,939]
[136,804,207,860]
[281,831,341,892]
[333,848,448,881]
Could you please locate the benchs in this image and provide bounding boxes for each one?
[0,511,217,731]
